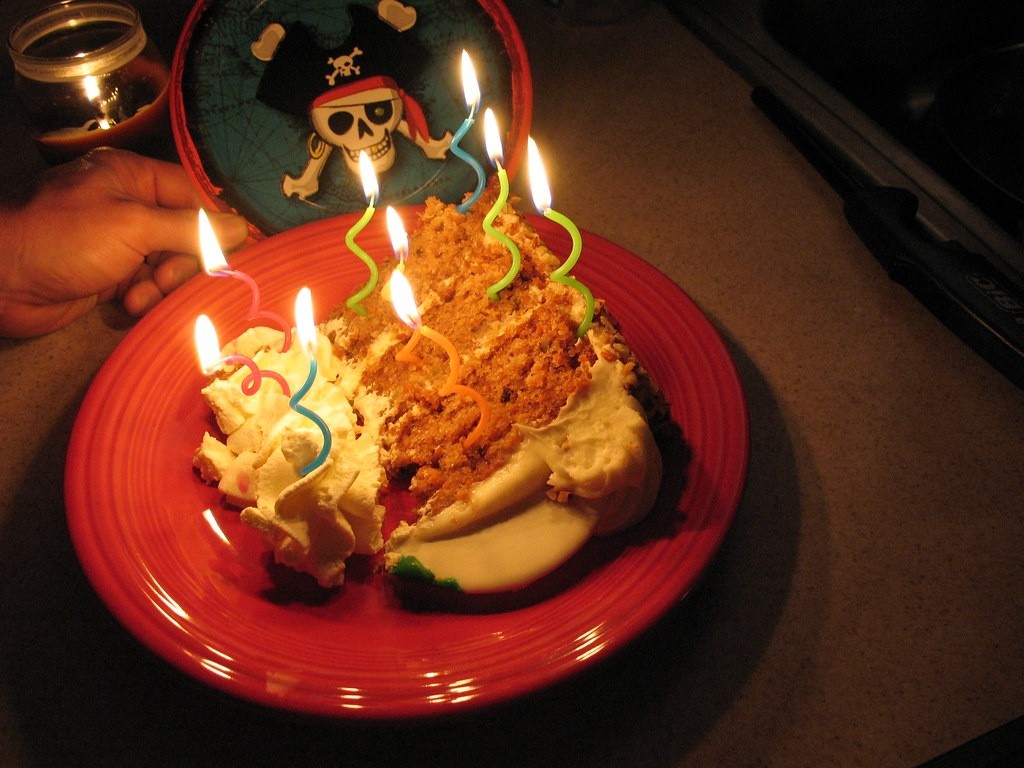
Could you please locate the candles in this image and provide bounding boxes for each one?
[288,282,334,476]
[481,106,522,302]
[194,314,294,399]
[525,134,594,338]
[391,267,489,448]
[343,149,380,315]
[196,207,292,352]
[380,205,426,372]
[448,50,485,213]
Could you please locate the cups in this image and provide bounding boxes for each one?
[6,0,169,154]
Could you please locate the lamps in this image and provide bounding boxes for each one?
[7,0,174,166]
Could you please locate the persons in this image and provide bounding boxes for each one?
[0,146,247,340]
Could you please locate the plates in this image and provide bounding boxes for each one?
[63,205,747,714]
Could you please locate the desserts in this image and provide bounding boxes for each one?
[195,196,657,604]
